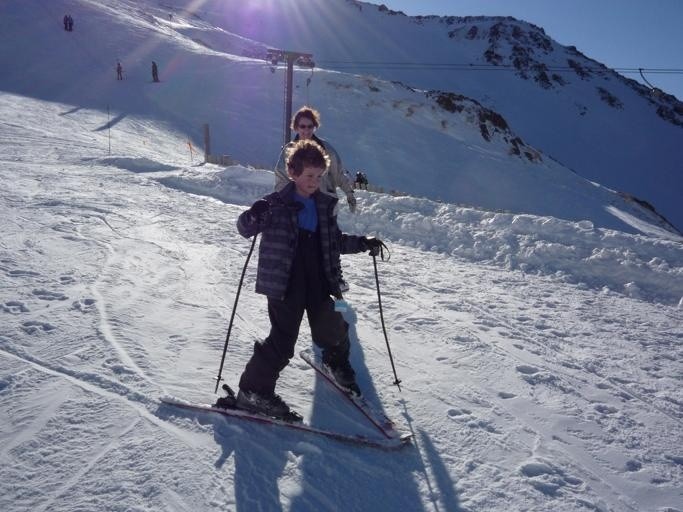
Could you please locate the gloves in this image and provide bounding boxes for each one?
[347,195,357,214]
[363,237,381,257]
[251,199,271,219]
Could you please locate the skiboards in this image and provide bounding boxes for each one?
[157,350,412,450]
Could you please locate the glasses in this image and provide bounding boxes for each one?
[297,124,315,130]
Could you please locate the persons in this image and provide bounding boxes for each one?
[63,13,68,31]
[235,139,382,418]
[115,61,122,80]
[353,171,368,190]
[343,169,351,183]
[272,105,357,220]
[67,13,73,31]
[150,60,159,82]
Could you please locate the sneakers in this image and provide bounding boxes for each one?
[236,387,290,417]
[322,348,356,391]
